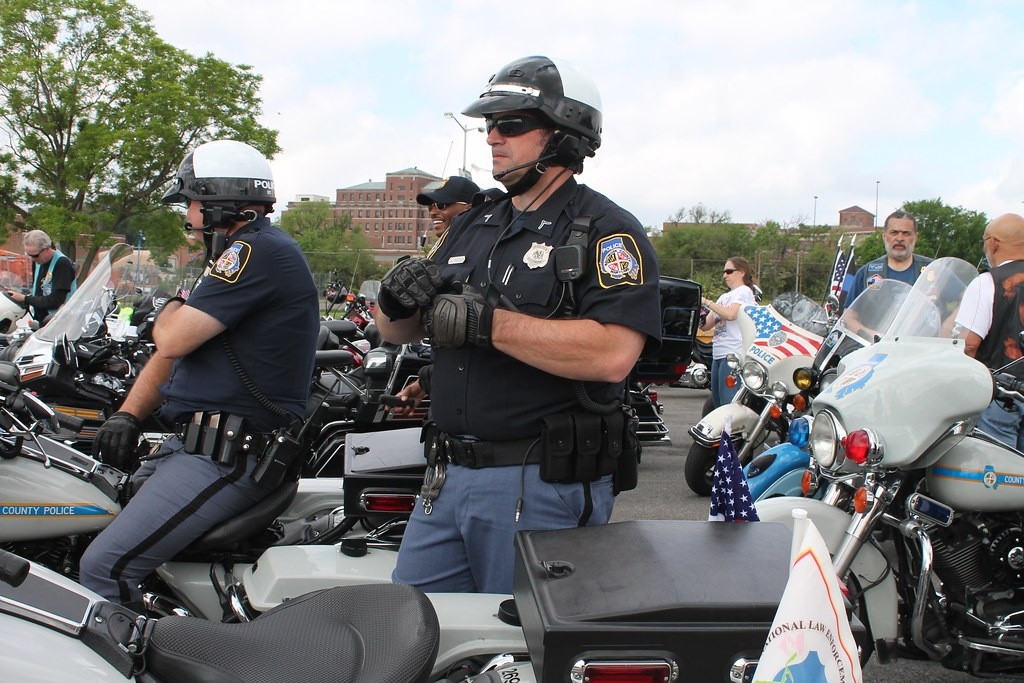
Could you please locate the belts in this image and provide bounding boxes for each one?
[439,434,543,469]
[237,433,268,456]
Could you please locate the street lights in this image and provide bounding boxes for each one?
[875,181,880,231]
[445,112,485,172]
[813,196,818,226]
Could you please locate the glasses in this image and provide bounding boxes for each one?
[485,115,555,137]
[428,201,469,212]
[28,249,45,258]
[183,195,191,208]
[723,268,738,275]
[980,236,1000,247]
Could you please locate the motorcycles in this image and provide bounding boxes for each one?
[0,518,859,683]
[0,266,1024,576]
[731,255,1024,682]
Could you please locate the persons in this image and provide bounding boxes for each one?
[954,213,1024,454]
[376,55,663,594]
[843,210,967,343]
[8,229,77,323]
[80,140,321,617]
[701,256,756,408]
[384,175,481,417]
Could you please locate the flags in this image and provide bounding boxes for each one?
[831,249,857,316]
[751,519,863,683]
[708,431,760,522]
[181,283,190,300]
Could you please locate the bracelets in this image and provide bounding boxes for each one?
[707,301,713,308]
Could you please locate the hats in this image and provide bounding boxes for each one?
[415,176,480,205]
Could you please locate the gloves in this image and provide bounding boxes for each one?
[378,254,444,323]
[420,279,494,352]
[130,287,186,343]
[91,411,142,475]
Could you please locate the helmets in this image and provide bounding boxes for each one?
[461,55,604,158]
[161,140,277,203]
